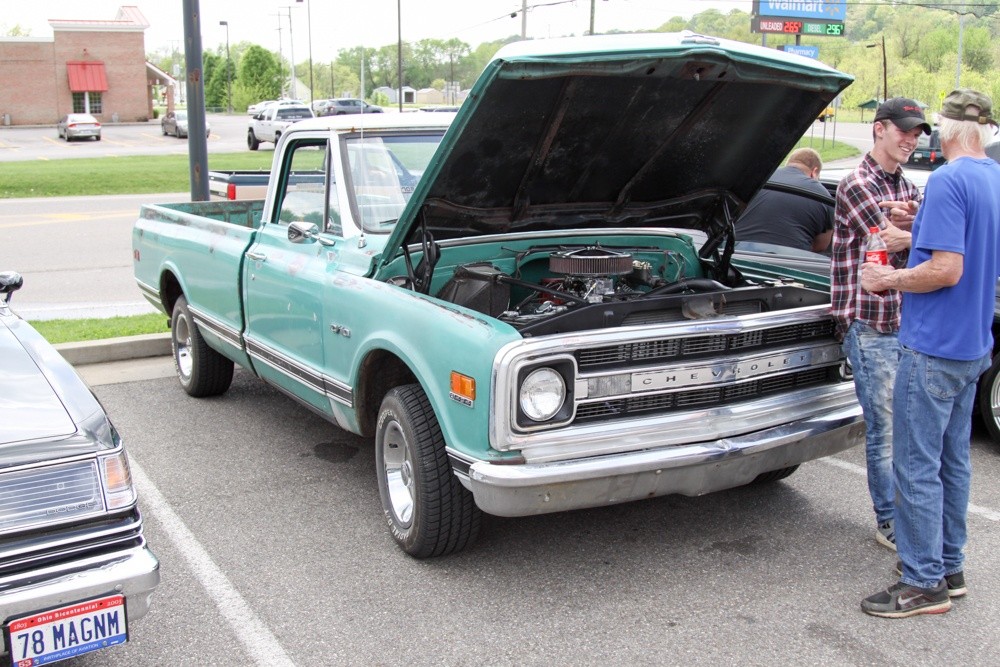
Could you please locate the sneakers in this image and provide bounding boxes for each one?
[861,577,952,618]
[876,517,897,550]
[896,559,967,596]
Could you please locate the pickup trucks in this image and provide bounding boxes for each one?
[906,128,946,166]
[133,30,870,559]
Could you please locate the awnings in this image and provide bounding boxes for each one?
[66,61,108,91]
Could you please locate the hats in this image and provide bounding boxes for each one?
[941,88,999,136]
[874,96,932,136]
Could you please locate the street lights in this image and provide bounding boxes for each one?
[217,21,233,115]
[868,35,887,103]
[296,0,314,106]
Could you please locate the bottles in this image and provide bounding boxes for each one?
[865,226,889,297]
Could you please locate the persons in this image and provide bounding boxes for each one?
[828,96,933,551]
[861,88,1000,619]
[734,146,836,253]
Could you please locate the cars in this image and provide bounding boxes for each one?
[161,110,211,139]
[59,112,103,141]
[0,273,161,662]
[817,175,1000,446]
[245,98,385,150]
